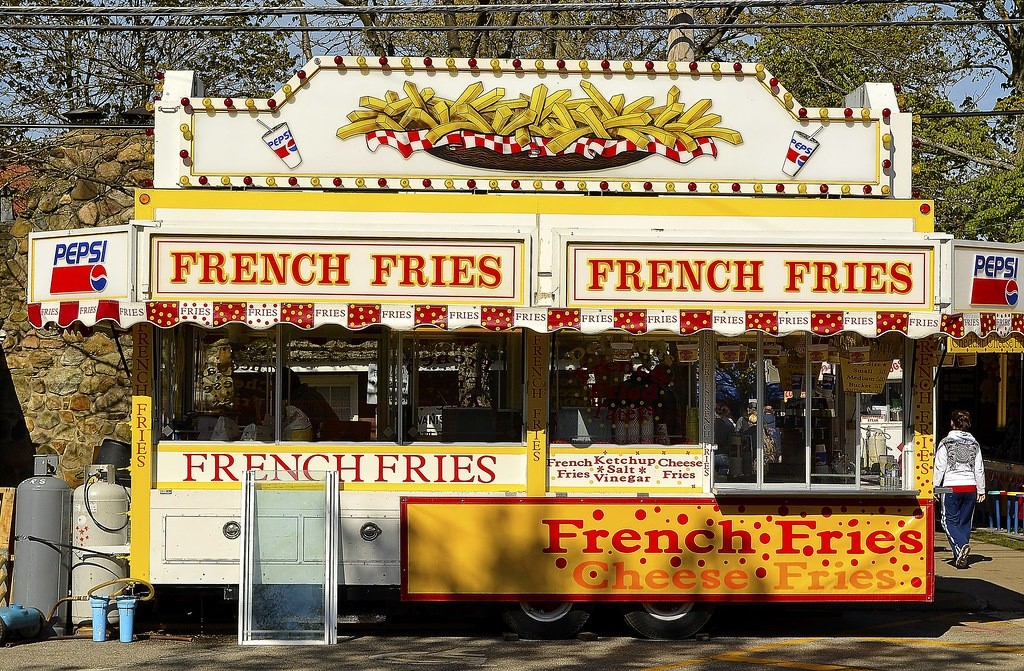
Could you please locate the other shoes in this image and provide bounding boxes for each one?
[955,543,970,569]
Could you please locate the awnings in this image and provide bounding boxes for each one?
[24,210,1024,340]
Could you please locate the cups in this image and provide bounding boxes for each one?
[737,346,747,362]
[809,344,829,363]
[719,346,739,363]
[611,343,632,361]
[850,347,869,364]
[678,345,698,362]
[616,408,667,436]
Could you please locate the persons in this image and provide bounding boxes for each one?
[713,401,783,482]
[270,366,344,439]
[933,407,986,570]
